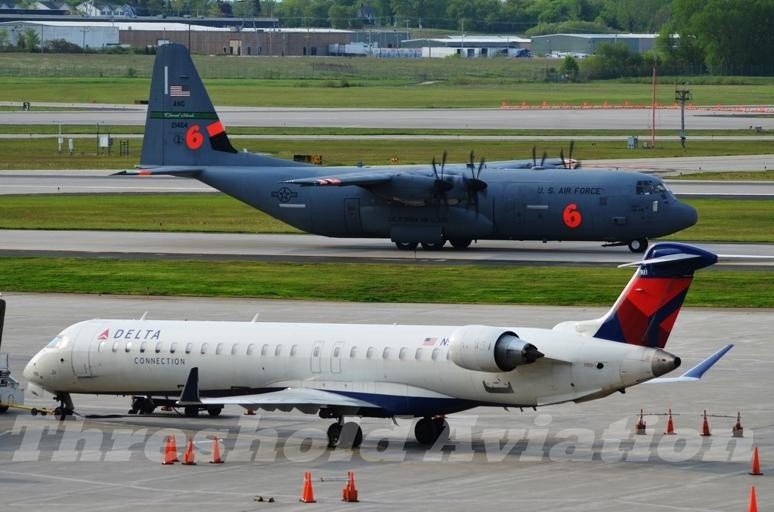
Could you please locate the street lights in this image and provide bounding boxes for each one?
[674,90,690,136]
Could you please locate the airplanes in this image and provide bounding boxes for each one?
[23,239,736,450]
[102,43,702,255]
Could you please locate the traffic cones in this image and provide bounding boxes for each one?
[663,409,679,435]
[733,410,745,438]
[699,409,714,436]
[162,434,180,465]
[298,471,316,503]
[208,435,224,464]
[636,409,650,436]
[181,438,199,465]
[747,487,760,511]
[341,471,360,502]
[748,447,766,475]
